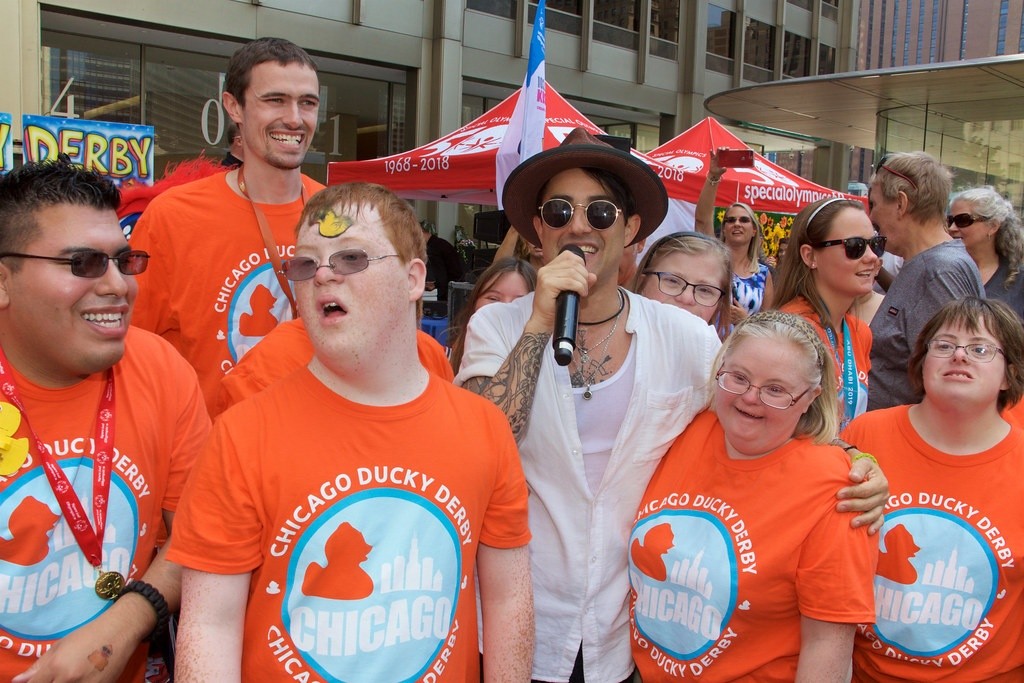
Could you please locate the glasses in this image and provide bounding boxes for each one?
[642,271,725,307]
[947,213,991,229]
[723,216,750,223]
[537,199,622,231]
[715,361,811,410]
[1,249,151,279]
[814,235,887,259]
[276,249,399,282]
[926,340,1005,363]
[876,157,917,190]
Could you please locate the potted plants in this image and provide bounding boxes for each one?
[456,239,474,270]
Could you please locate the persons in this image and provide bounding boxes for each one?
[0,37,1024,683]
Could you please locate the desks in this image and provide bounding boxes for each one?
[421,289,437,317]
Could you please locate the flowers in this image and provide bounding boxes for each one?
[717,209,797,271]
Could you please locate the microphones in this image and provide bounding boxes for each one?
[552,244,585,366]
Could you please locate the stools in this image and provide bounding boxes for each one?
[421,311,450,346]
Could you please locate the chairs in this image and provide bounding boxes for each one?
[448,281,476,337]
[467,210,512,271]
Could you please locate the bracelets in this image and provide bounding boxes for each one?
[831,436,842,447]
[850,453,880,468]
[842,445,859,453]
[114,580,168,640]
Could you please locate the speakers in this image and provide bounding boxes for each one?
[472,210,510,277]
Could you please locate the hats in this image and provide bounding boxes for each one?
[502,127,668,249]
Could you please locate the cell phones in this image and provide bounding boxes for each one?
[718,150,754,168]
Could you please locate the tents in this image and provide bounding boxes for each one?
[325,71,715,260]
[643,115,871,215]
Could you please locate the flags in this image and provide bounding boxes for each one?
[493,0,550,212]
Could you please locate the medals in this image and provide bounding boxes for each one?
[94,571,127,601]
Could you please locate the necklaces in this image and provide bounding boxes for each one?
[573,287,621,363]
[573,287,624,400]
[573,288,626,325]
